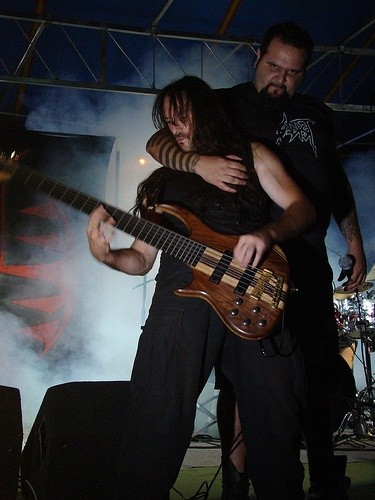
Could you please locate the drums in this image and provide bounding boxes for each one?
[364,298,375,331]
[341,308,371,340]
[334,307,348,336]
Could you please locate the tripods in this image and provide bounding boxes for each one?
[333,339,375,447]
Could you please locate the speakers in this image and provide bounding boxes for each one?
[0,385,23,500]
[21,380,131,500]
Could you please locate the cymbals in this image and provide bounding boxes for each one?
[365,265,375,280]
[334,282,374,294]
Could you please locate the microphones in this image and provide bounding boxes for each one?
[338,256,358,292]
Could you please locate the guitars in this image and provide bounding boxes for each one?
[0,151,299,342]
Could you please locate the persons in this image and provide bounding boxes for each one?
[86,75,319,500]
[145,22,368,500]
